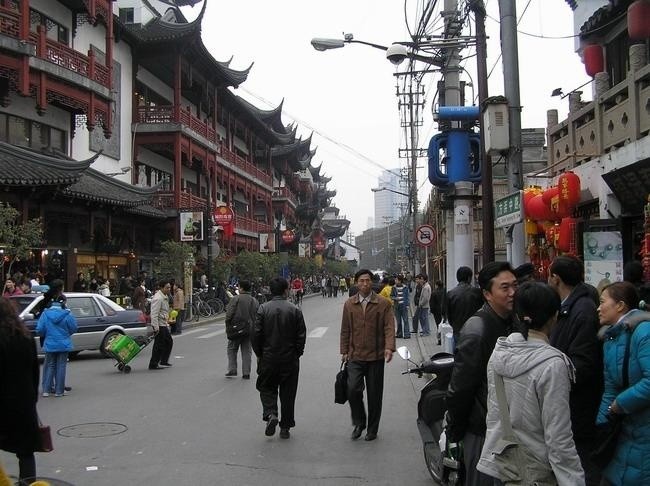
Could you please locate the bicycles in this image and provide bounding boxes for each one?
[123,282,327,321]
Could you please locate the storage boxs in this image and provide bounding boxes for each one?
[106,333,141,364]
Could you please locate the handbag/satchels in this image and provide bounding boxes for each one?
[493,436,558,486]
[335,361,347,404]
[588,411,623,470]
[439,318,455,354]
[226,317,250,339]
[34,414,53,452]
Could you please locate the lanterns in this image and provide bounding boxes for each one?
[584,44,604,77]
[523,171,580,252]
[627,1,650,41]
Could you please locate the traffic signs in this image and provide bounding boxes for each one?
[493,189,523,228]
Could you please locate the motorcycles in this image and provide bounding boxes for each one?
[397,345,467,486]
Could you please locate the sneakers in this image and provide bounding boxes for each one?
[224,370,250,379]
[42,387,71,397]
[265,413,290,438]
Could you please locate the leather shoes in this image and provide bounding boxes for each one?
[352,425,378,441]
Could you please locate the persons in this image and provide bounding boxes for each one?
[71,270,153,320]
[0,296,40,485]
[251,275,305,437]
[320,273,339,298]
[2,273,47,299]
[439,253,650,485]
[35,278,77,397]
[339,269,396,441]
[148,279,185,369]
[392,271,447,346]
[226,279,261,378]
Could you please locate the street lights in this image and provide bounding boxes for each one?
[370,185,413,211]
[383,219,413,244]
[313,38,463,132]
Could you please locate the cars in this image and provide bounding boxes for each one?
[371,271,388,282]
[7,293,148,361]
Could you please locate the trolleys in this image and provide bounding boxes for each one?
[108,329,160,375]
